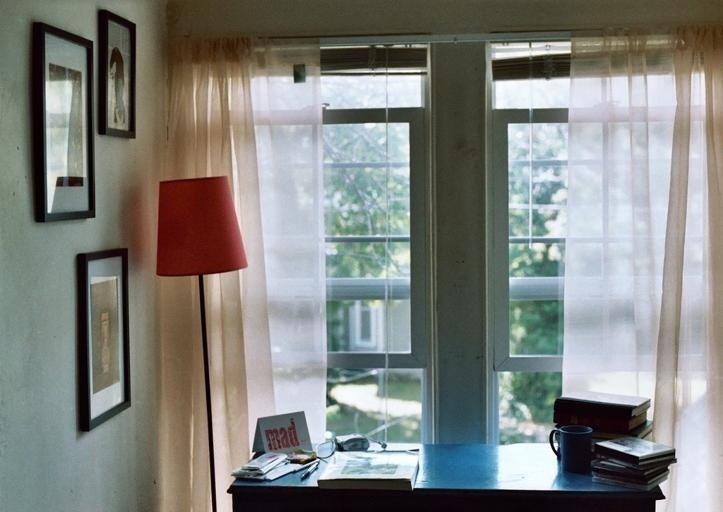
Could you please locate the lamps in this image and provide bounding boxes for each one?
[156,176,249,512]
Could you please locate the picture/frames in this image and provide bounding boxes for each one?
[75,247,131,431]
[30,20,96,222]
[97,9,137,139]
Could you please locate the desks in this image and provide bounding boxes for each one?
[227,442,665,512]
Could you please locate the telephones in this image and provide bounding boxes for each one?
[300,461,319,480]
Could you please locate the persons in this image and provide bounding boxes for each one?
[93,309,119,390]
[109,46,129,130]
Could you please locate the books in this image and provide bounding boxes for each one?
[552,390,679,492]
[316,451,420,492]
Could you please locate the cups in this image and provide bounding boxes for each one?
[548,424,593,474]
